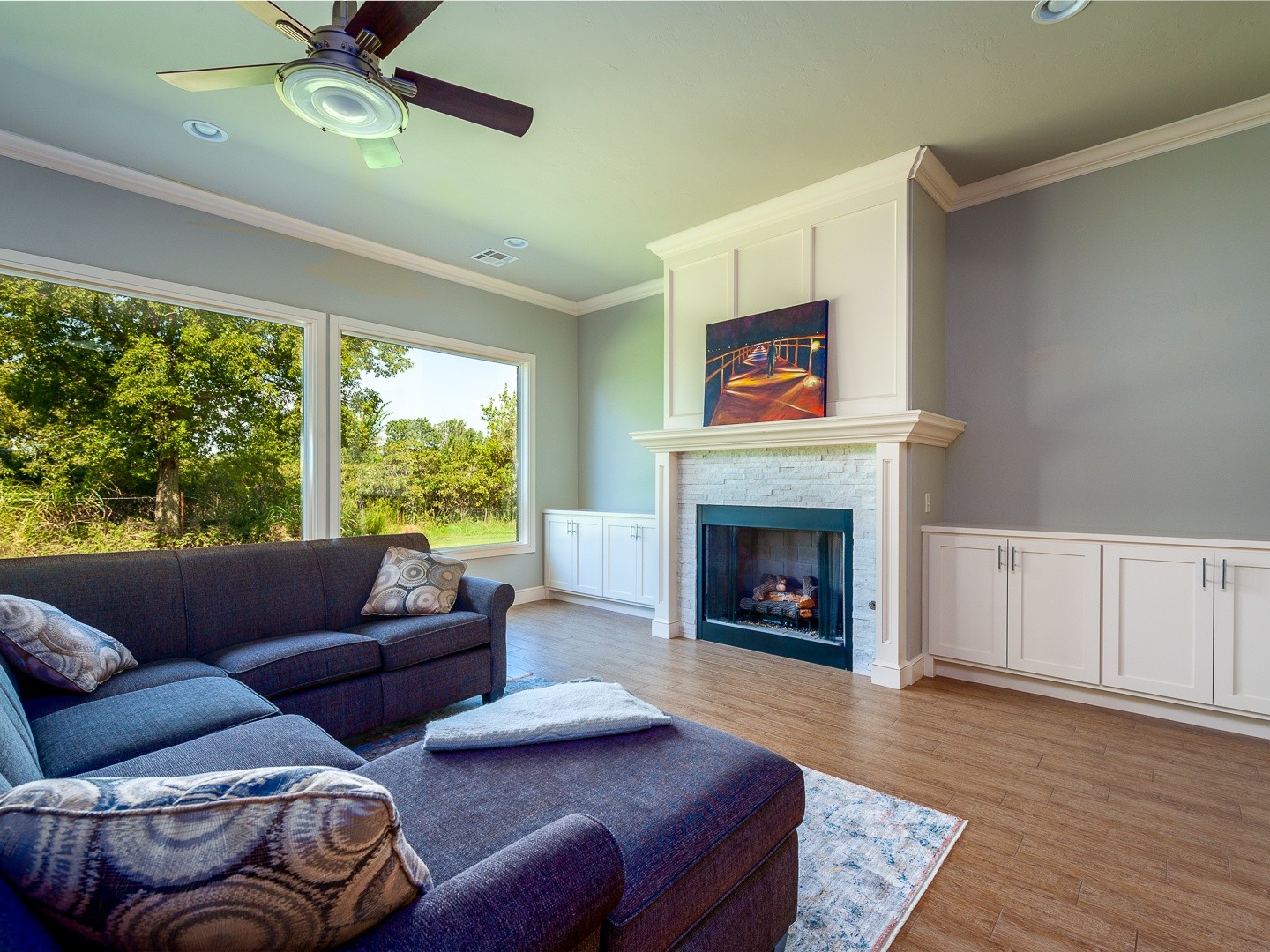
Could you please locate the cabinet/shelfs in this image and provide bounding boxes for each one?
[603,517,657,607]
[1102,544,1270,740]
[544,515,604,598]
[927,534,1101,707]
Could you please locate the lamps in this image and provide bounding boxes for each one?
[275,20,418,139]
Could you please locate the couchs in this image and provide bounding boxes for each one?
[0,534,805,952]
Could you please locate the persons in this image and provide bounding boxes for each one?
[766,339,778,378]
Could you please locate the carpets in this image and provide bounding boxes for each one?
[351,673,968,952]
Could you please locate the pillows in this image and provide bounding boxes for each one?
[0,764,434,952]
[0,594,138,694]
[361,545,468,616]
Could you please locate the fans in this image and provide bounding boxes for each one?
[156,0,534,169]
